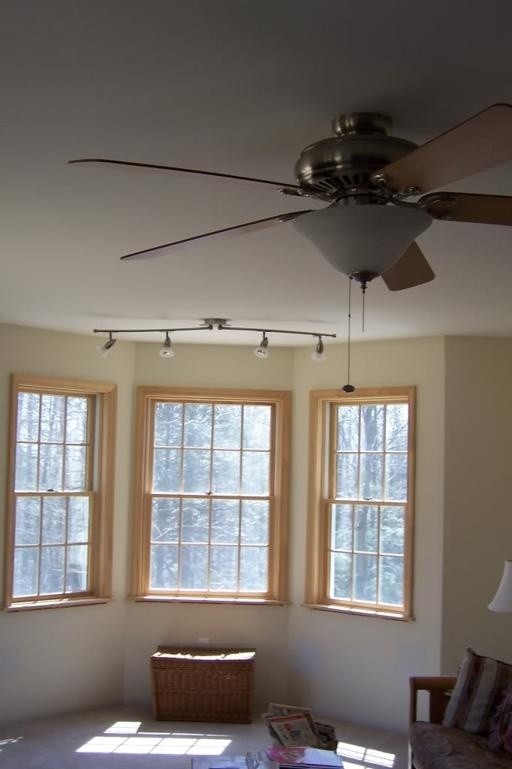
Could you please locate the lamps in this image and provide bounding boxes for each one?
[95,330,326,364]
[293,198,434,290]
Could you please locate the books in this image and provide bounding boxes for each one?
[258,703,343,769]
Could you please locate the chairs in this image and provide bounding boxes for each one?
[408,675,512,769]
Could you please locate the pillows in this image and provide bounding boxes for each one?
[440,643,511,756]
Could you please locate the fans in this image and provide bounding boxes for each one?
[65,98,512,294]
